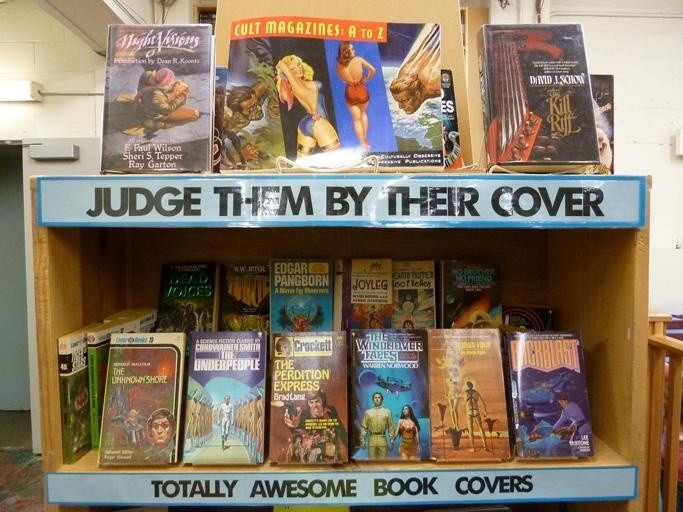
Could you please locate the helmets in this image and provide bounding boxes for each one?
[304,387,327,409]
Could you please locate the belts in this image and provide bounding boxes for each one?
[370,431,386,436]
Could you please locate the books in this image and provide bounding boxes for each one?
[57,257,595,468]
[100,18,614,176]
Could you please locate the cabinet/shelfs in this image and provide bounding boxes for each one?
[1,79,43,103]
[648,313,682,512]
[30,172,651,512]
